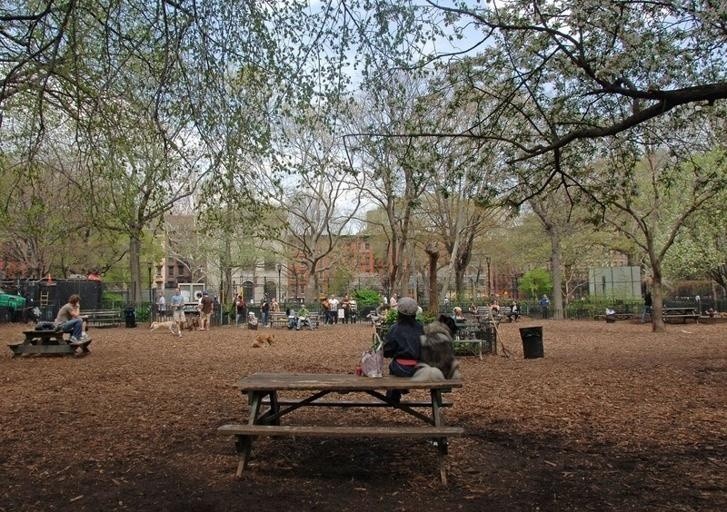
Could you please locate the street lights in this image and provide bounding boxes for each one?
[147,262,152,305]
[486,256,491,296]
[277,264,281,303]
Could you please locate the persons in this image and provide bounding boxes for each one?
[382,295,388,312]
[259,296,313,331]
[452,305,465,319]
[507,300,521,322]
[32,305,42,323]
[196,291,219,331]
[389,292,398,310]
[381,297,424,402]
[155,291,166,321]
[170,288,186,338]
[235,296,244,327]
[54,294,83,343]
[322,294,357,324]
[540,293,550,319]
[469,301,480,323]
[641,292,652,322]
[490,299,500,325]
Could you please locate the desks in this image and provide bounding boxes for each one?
[12,330,77,358]
[231,372,462,485]
[661,308,699,324]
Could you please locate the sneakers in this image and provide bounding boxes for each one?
[70,334,88,343]
[386,390,401,403]
[178,332,183,338]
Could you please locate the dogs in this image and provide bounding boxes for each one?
[150,320,183,338]
[251,333,275,350]
[409,314,462,383]
[176,317,199,332]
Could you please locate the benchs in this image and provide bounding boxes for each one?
[471,306,521,323]
[217,400,465,486]
[80,309,121,326]
[267,312,321,329]
[662,314,701,324]
[7,338,93,359]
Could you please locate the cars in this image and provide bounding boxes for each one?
[0,287,26,322]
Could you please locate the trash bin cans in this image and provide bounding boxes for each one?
[46,305,57,322]
[518,326,544,360]
[124,306,135,328]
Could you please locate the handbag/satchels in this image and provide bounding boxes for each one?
[360,332,385,378]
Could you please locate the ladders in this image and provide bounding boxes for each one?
[40,291,49,308]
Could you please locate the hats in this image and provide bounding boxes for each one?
[397,297,418,315]
